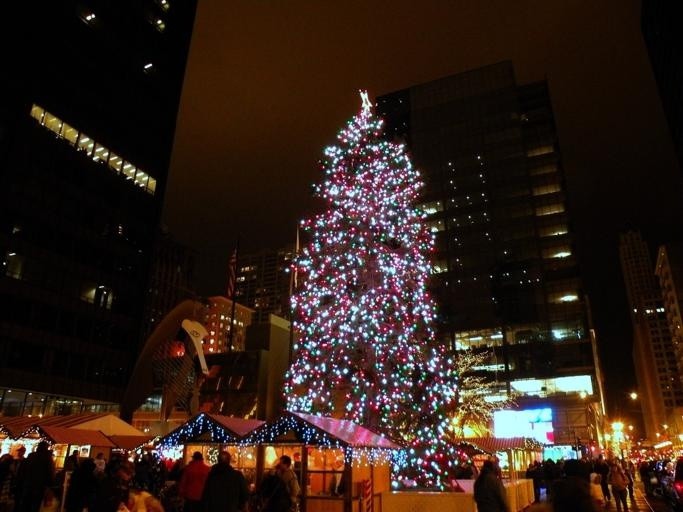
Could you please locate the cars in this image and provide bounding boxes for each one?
[639,456,682,511]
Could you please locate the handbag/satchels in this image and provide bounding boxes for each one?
[614,479,625,491]
[594,476,600,484]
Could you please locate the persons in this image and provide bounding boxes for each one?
[0,440,309,511]
[471,453,683,512]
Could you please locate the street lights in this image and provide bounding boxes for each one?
[612,421,626,460]
